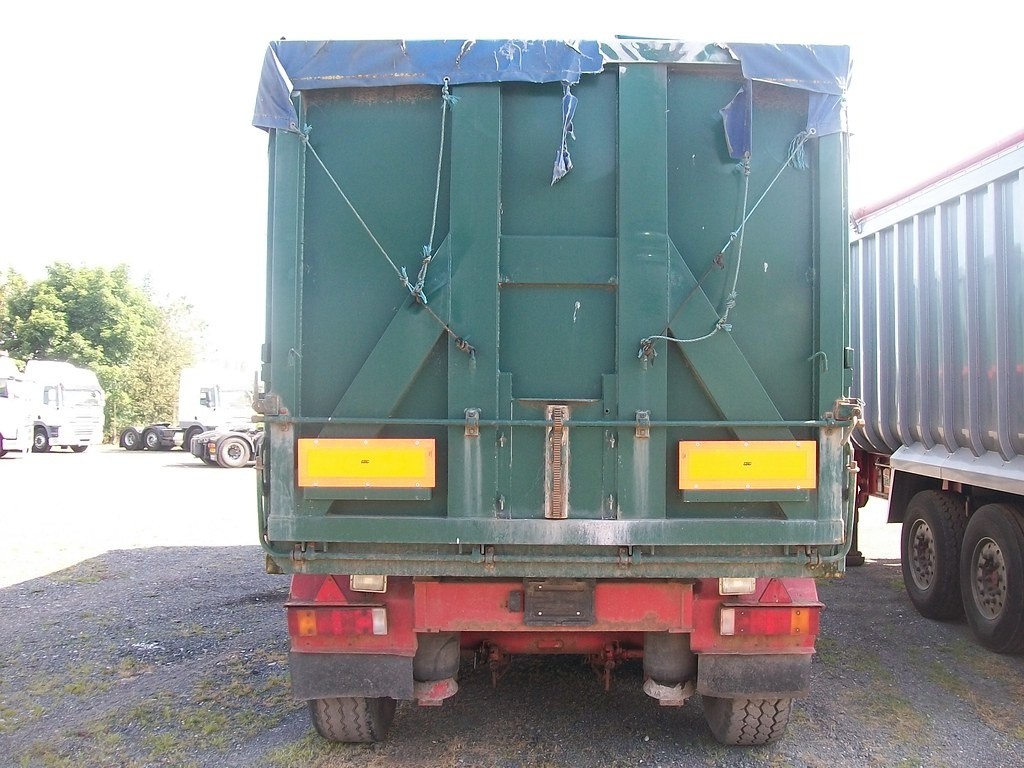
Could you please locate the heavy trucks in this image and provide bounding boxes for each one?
[120,366,254,452]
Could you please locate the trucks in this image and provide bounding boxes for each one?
[0,355,106,453]
[251,31,860,751]
